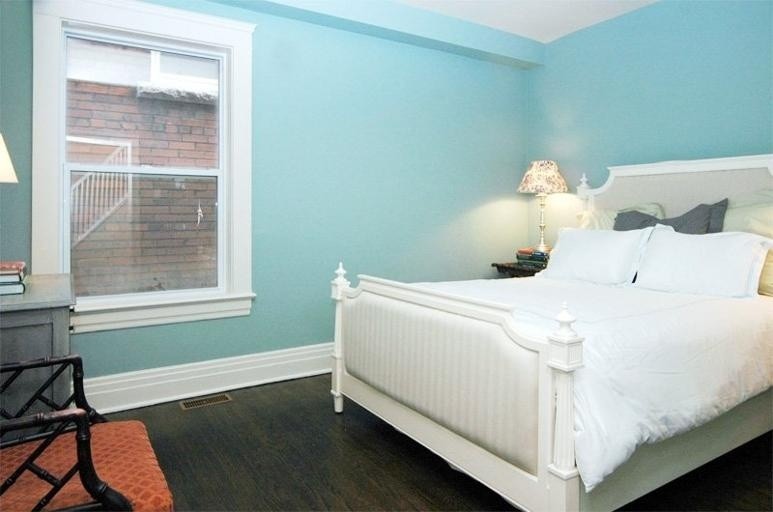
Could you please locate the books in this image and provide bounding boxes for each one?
[518,249,549,267]
[0,262,25,295]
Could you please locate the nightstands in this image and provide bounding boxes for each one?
[490,261,543,278]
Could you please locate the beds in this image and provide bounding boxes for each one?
[330,153,772,512]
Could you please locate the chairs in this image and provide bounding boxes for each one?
[3,354,175,512]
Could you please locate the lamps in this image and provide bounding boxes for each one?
[517,160,568,252]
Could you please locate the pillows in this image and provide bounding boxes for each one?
[540,191,772,297]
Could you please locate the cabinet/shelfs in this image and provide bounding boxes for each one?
[1,274,78,445]
